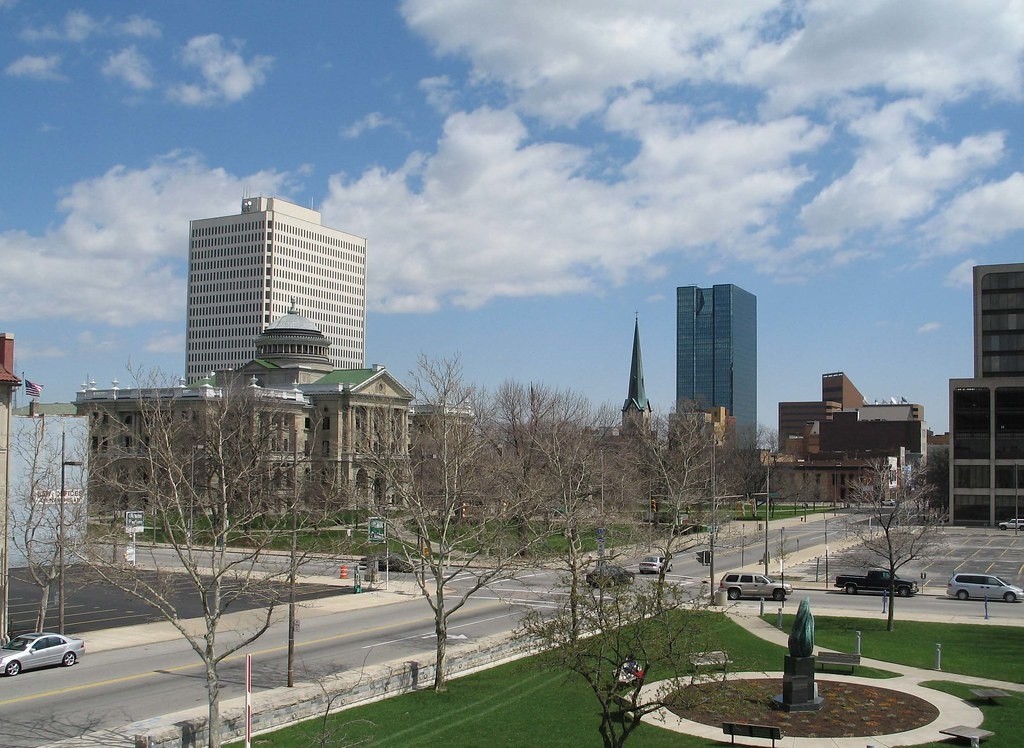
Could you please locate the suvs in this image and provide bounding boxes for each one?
[719,571,794,601]
[998,518,1024,531]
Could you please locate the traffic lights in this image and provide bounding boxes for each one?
[686,505,690,515]
[650,499,656,513]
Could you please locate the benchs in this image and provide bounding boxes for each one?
[612,693,633,710]
[689,651,733,672]
[612,668,638,687]
[815,651,861,674]
[722,722,785,748]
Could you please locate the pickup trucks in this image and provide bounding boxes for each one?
[833,569,919,598]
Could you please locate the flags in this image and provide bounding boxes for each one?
[24,380,44,397]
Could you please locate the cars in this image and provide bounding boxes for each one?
[360,552,418,573]
[638,556,673,574]
[0,632,86,677]
[946,573,1024,603]
[881,498,896,506]
[586,564,637,588]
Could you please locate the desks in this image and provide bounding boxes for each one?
[939,725,995,740]
[968,689,1012,700]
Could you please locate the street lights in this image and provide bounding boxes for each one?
[188,445,205,565]
[417,453,437,549]
[834,462,843,516]
[57,430,84,633]
